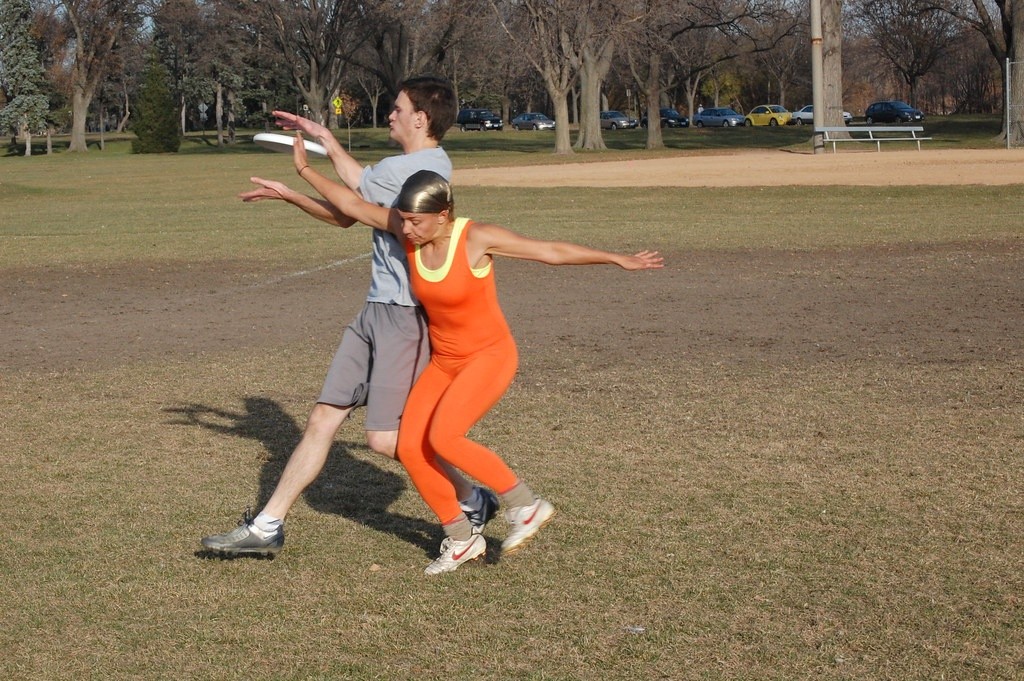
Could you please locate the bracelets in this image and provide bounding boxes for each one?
[299,166,309,175]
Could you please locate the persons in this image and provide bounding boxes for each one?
[292,129,664,576]
[201,77,500,553]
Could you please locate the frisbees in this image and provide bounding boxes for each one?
[253,133,327,157]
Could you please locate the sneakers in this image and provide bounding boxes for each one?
[463,486,500,535]
[424,527,487,576]
[501,498,558,555]
[201,509,285,557]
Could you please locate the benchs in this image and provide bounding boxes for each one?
[815,127,932,154]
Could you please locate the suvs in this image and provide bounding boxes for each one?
[456,108,503,133]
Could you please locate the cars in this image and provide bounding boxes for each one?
[742,104,792,128]
[865,100,925,126]
[511,112,556,131]
[790,104,853,126]
[640,107,689,129]
[600,111,639,131]
[691,107,745,128]
